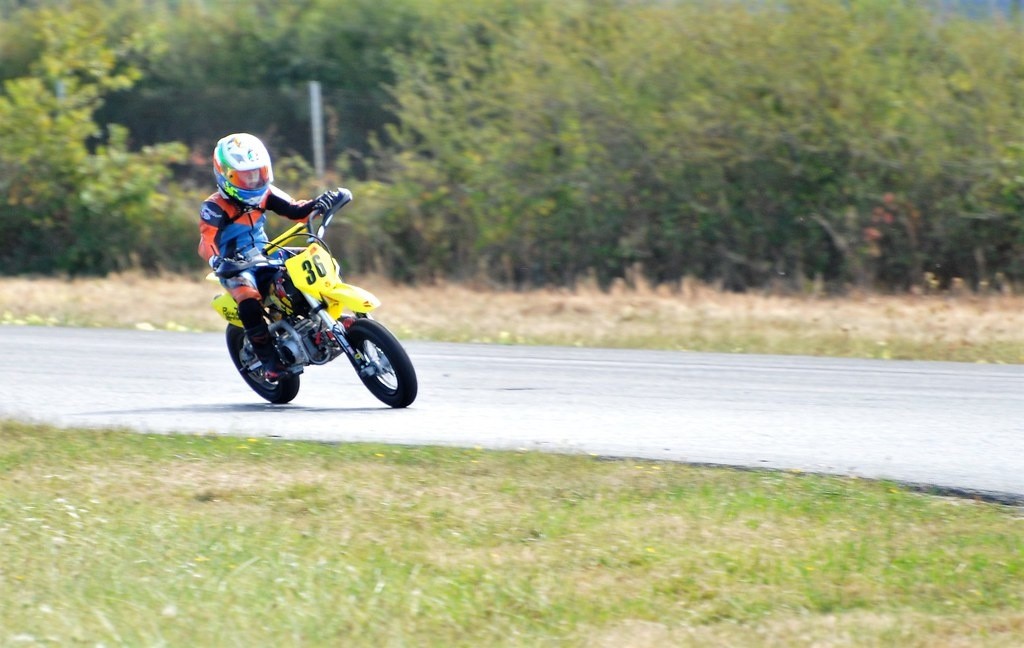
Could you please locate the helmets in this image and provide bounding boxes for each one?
[213,132,274,205]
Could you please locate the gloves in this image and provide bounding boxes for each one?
[212,256,236,271]
[312,190,340,215]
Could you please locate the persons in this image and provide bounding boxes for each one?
[198,133,332,379]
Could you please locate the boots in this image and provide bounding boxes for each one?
[245,325,288,378]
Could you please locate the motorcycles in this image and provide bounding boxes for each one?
[205,187,419,410]
